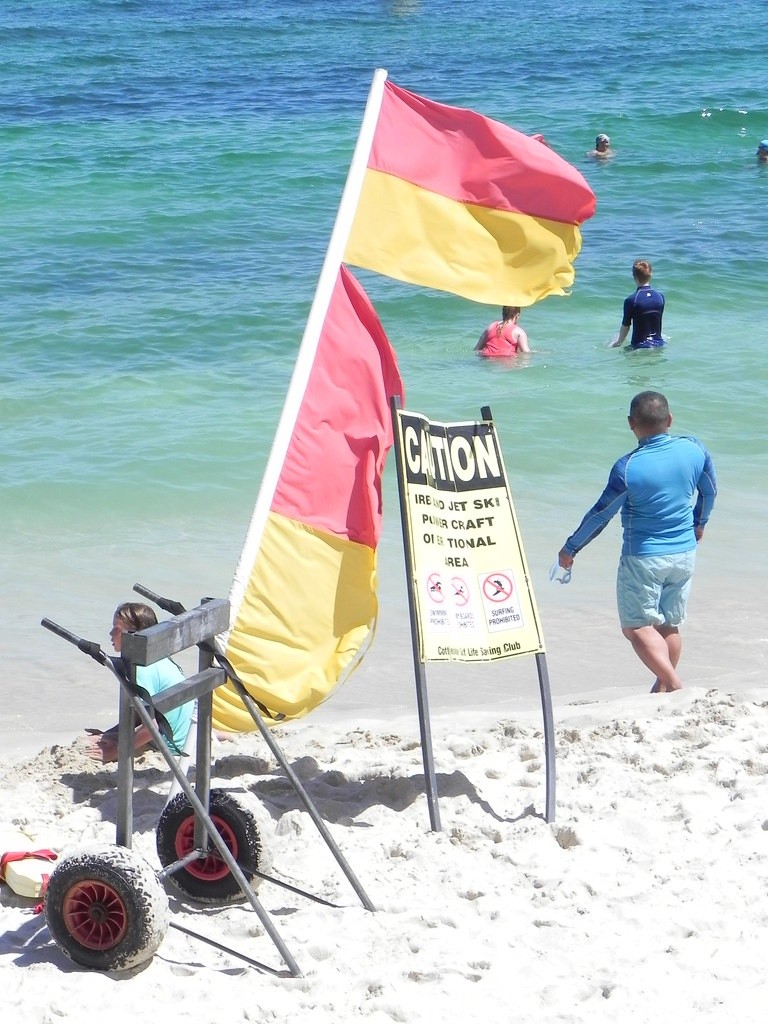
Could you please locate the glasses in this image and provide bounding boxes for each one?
[549,565,572,584]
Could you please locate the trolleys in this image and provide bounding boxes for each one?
[41,583,376,977]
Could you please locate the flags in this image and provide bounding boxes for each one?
[190,263,406,733]
[341,79,597,307]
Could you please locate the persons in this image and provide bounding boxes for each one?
[474,306,531,355]
[759,140,768,158]
[83,602,195,762]
[559,390,718,693]
[612,260,665,348]
[592,133,610,155]
